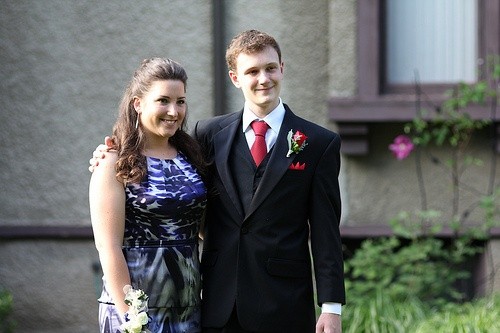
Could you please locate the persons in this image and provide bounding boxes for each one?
[88,57,209,333]
[88,29,346,333]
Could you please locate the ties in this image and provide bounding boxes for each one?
[250,120,270,168]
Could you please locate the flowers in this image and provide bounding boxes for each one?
[292,130,309,153]
